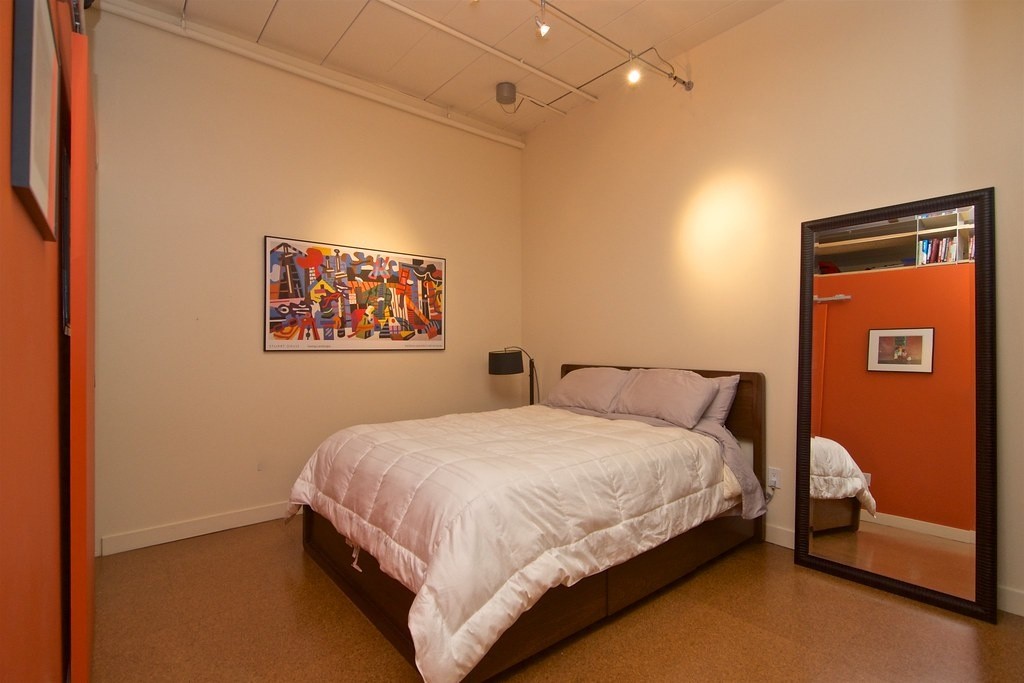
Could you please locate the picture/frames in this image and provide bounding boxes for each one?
[866,327,934,373]
[262,236,447,352]
[13,1,62,246]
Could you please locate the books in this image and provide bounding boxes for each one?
[918,233,976,263]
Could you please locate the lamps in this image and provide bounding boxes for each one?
[487,345,540,406]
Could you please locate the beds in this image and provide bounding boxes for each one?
[809,433,878,535]
[284,365,774,683]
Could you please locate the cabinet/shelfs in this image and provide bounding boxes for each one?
[813,206,976,281]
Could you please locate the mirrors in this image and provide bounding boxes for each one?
[793,184,998,628]
